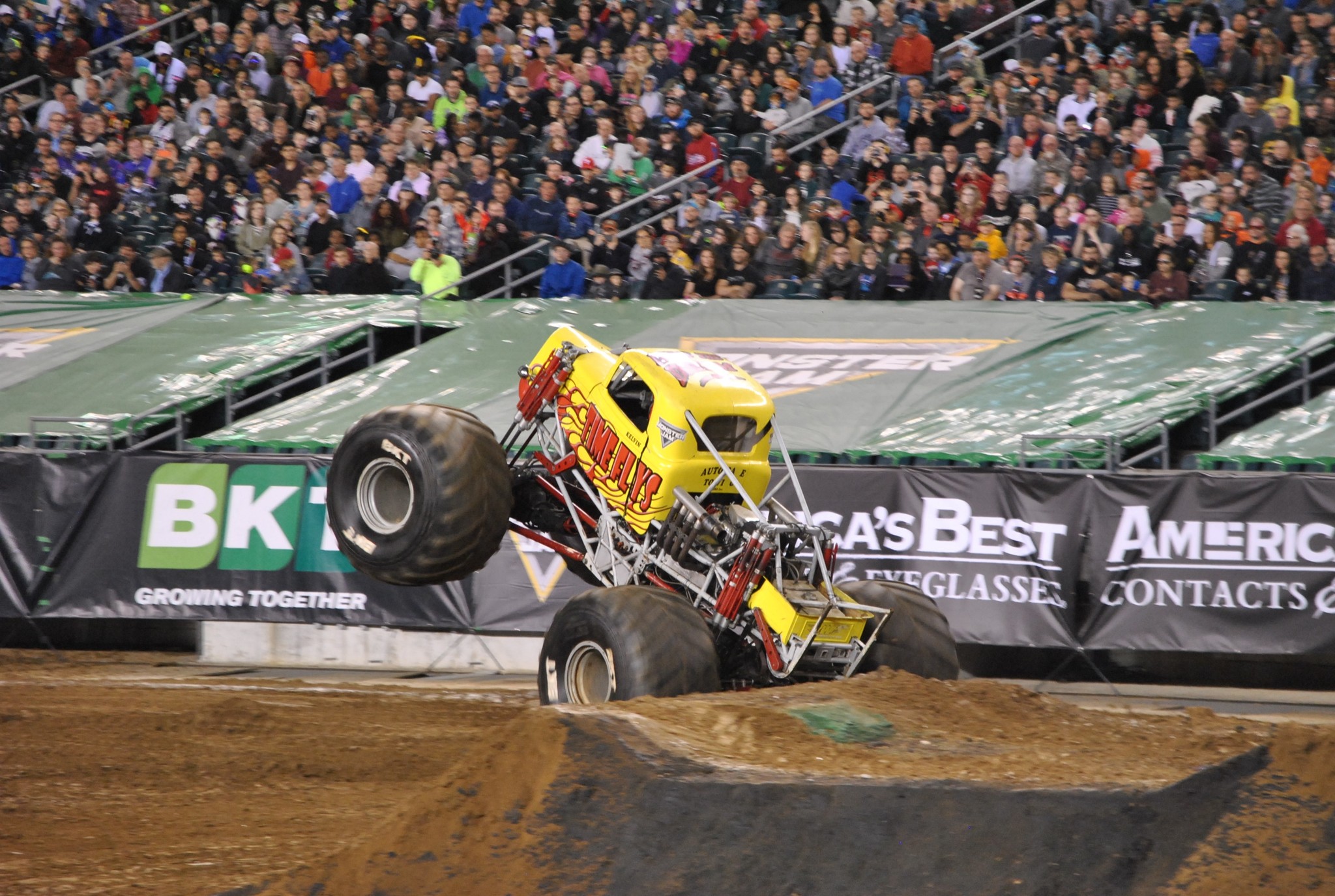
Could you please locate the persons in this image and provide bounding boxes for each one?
[0,0,1335,303]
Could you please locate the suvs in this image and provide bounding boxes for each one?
[326,322,960,708]
